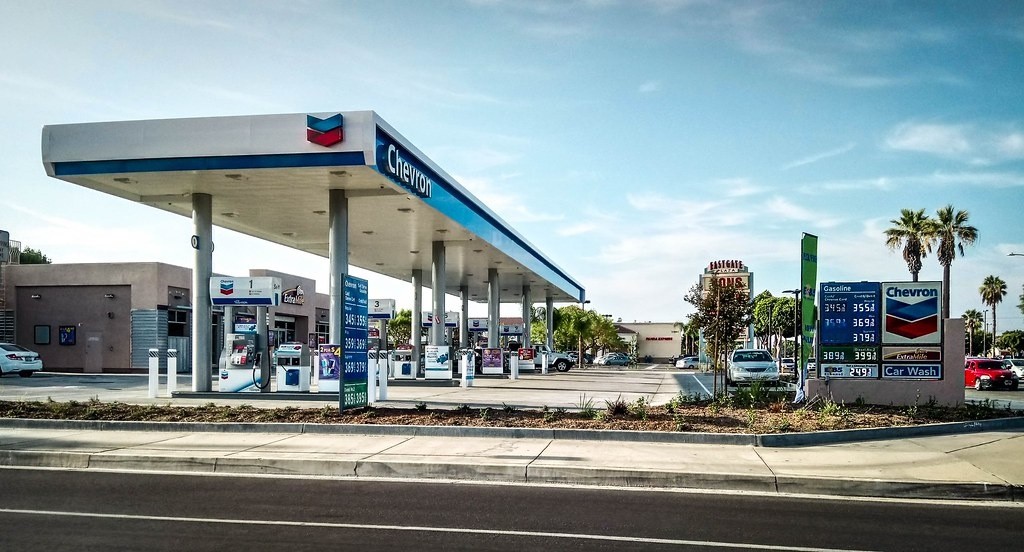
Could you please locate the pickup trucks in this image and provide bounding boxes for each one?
[502,344,575,372]
[668,354,695,367]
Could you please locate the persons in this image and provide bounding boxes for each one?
[644,355,648,363]
[710,356,712,362]
[632,355,637,365]
[706,354,710,362]
[671,355,674,359]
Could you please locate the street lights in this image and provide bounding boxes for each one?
[577,300,590,312]
[602,315,612,323]
[982,309,989,357]
[782,289,802,381]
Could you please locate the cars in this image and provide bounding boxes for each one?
[0,343,43,378]
[781,357,795,372]
[727,348,779,387]
[565,350,634,366]
[964,356,1024,391]
[676,356,699,370]
[807,358,815,371]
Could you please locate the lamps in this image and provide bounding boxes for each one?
[190,235,200,250]
[104,294,114,298]
[321,314,326,317]
[174,296,182,299]
[31,294,41,300]
